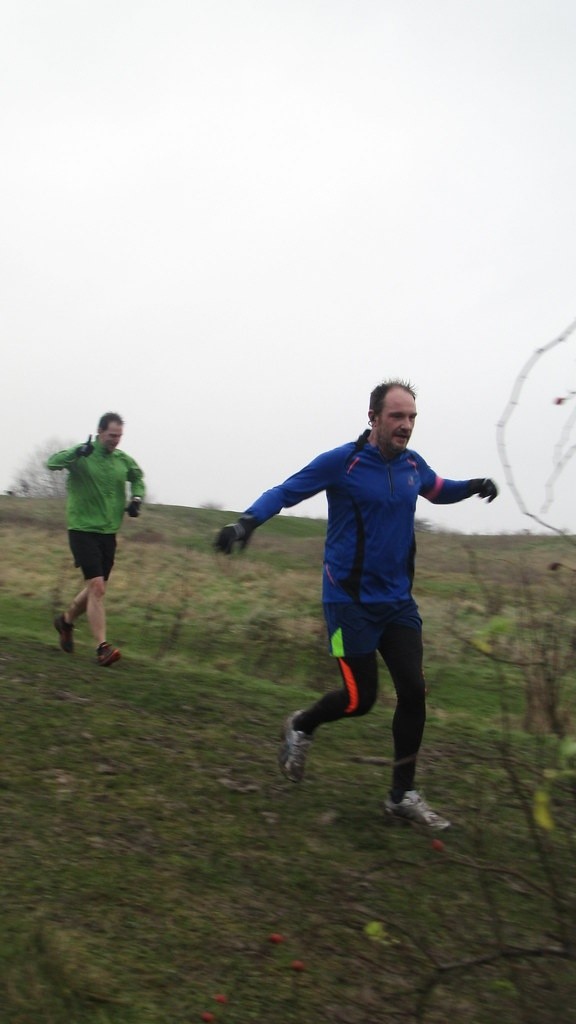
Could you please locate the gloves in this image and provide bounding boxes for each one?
[75,434,94,456]
[123,499,142,517]
[216,515,253,553]
[466,478,498,503]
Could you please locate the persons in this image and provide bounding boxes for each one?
[46,411,145,667]
[215,380,499,833]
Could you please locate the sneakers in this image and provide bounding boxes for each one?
[54,612,75,654]
[278,709,315,783]
[383,789,450,834]
[97,641,122,666]
